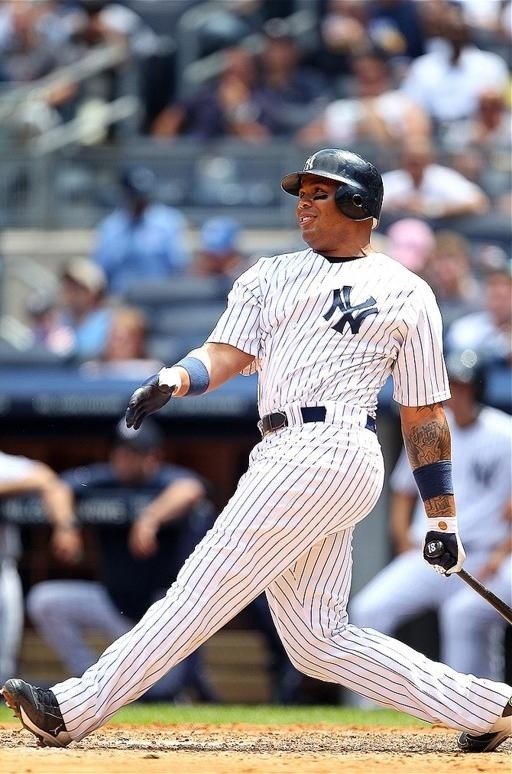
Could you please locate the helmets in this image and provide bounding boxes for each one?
[282,148,385,230]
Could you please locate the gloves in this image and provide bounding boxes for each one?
[122,365,181,434]
[420,527,467,580]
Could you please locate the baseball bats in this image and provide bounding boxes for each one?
[426,540,511,623]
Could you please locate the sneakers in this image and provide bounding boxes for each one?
[0,660,75,750]
[457,682,512,753]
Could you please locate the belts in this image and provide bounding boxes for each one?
[255,408,378,440]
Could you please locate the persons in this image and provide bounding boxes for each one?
[1,147,512,753]
[0,0,512,704]
